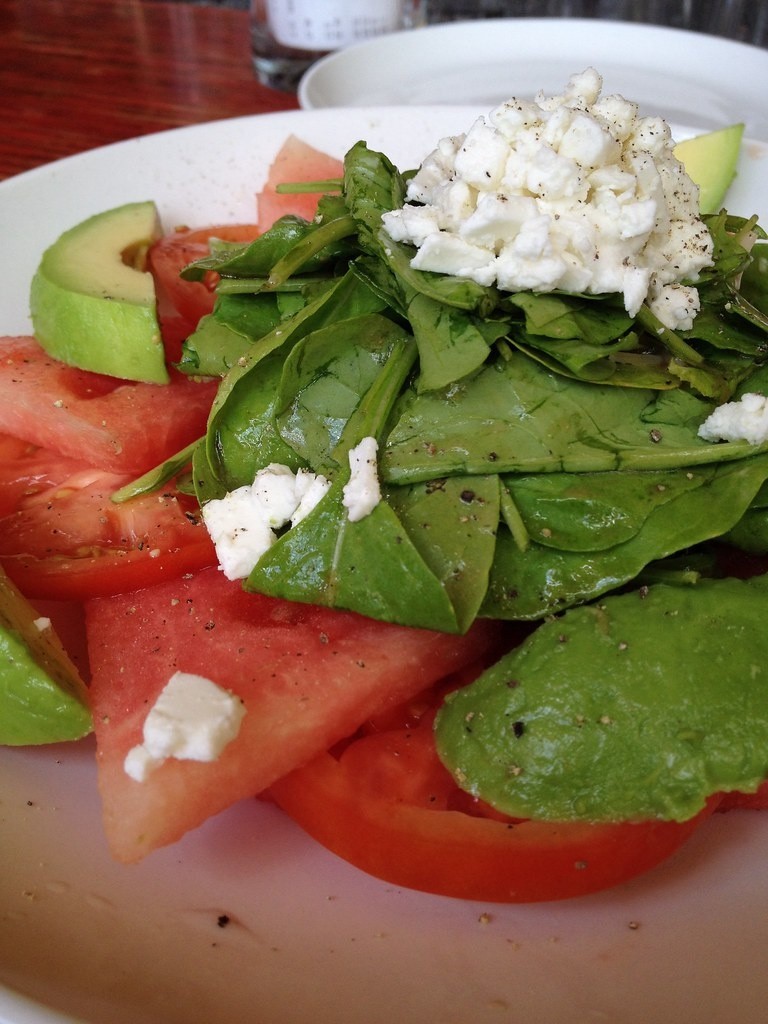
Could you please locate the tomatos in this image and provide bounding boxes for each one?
[2,132,768,901]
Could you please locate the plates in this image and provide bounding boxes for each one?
[6,94,764,1024]
[297,15,767,151]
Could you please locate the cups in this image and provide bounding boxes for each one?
[247,0,427,93]
